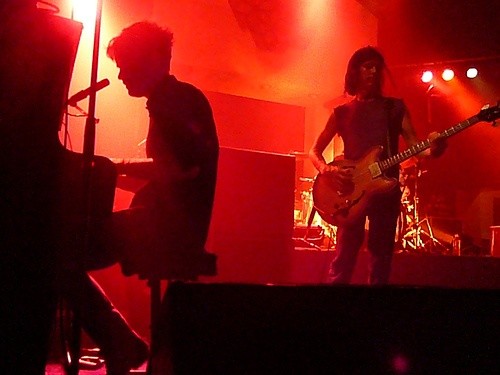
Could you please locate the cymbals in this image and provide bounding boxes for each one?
[299,177,314,182]
[290,151,310,159]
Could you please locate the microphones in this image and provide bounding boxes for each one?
[70,79,109,104]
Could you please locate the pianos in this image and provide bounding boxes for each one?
[0,0,118,375]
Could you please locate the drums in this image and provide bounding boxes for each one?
[299,190,337,236]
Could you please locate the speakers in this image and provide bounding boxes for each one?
[146,279,500,375]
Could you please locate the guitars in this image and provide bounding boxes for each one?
[312,102,500,227]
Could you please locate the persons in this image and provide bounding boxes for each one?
[308,46,446,284]
[44,21,220,375]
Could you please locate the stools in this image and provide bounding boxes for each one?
[121,252,217,375]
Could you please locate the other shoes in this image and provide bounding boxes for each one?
[103,332,148,375]
